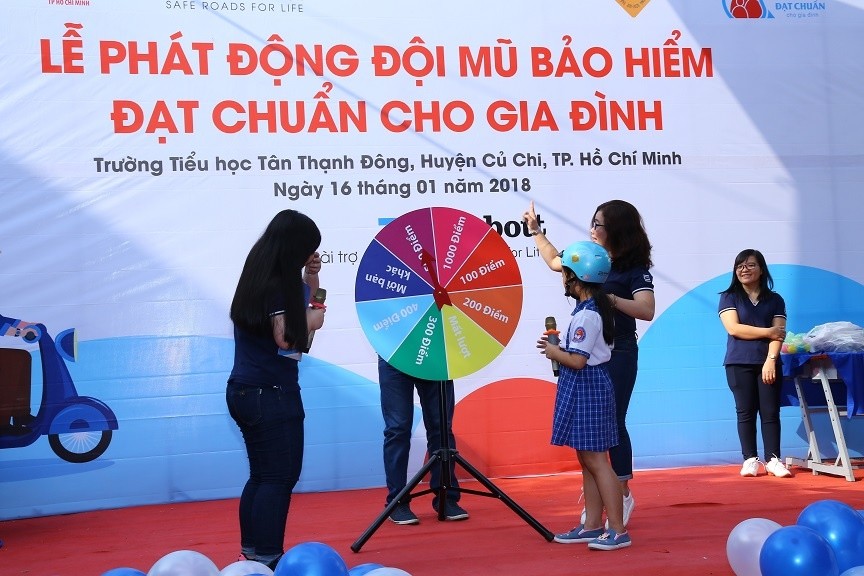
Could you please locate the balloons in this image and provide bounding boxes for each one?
[100,542,413,576]
[726,500,864,576]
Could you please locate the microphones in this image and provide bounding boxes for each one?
[305,288,326,354]
[545,317,559,377]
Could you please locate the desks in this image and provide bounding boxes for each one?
[776,351,864,481]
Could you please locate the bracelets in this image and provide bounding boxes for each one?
[305,266,319,274]
[611,293,617,305]
[528,226,541,235]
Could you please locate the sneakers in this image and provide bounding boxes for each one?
[765,453,792,478]
[588,527,632,551]
[442,503,469,521]
[554,523,605,544]
[740,456,764,477]
[604,491,634,531]
[578,486,586,525]
[387,503,420,525]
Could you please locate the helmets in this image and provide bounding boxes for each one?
[561,240,612,284]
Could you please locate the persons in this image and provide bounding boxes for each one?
[718,248,792,477]
[522,199,656,532]
[378,354,471,525]
[535,240,633,552]
[225,209,326,568]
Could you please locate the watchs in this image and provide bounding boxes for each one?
[767,355,776,360]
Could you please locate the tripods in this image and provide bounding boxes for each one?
[350,380,555,555]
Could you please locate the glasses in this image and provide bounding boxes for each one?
[736,262,757,271]
[593,222,606,229]
